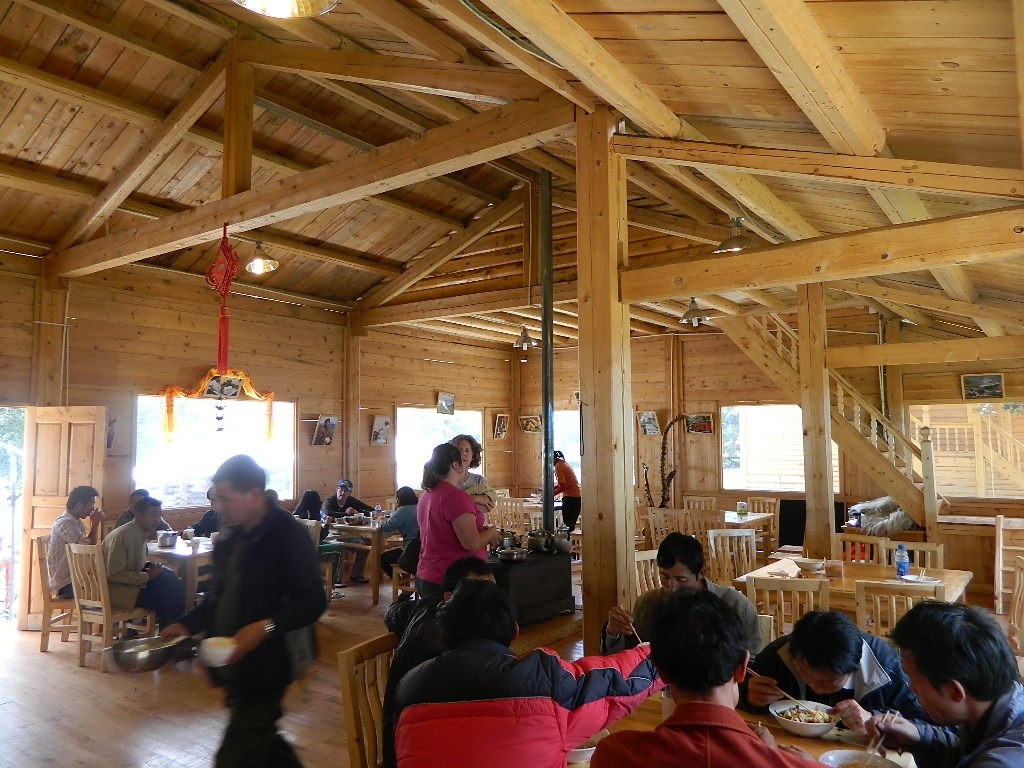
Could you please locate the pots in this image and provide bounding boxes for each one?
[489,528,520,554]
[491,544,533,560]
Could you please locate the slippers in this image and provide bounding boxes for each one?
[398,590,414,601]
[349,575,369,583]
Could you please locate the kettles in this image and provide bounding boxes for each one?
[527,527,555,553]
[158,529,178,547]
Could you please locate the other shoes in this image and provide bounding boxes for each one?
[334,581,347,587]
[332,591,345,598]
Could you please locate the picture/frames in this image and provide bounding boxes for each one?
[436,391,455,416]
[636,411,661,437]
[518,415,543,433]
[203,374,243,400]
[370,415,391,447]
[961,371,1006,400]
[682,413,714,434]
[311,413,339,447]
[493,413,510,440]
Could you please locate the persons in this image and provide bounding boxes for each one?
[159,452,331,768]
[371,530,1024,768]
[317,418,333,444]
[47,435,576,661]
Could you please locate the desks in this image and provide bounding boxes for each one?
[326,522,403,605]
[485,500,563,531]
[640,501,775,562]
[730,557,973,638]
[842,523,926,568]
[561,691,917,768]
[146,536,215,615]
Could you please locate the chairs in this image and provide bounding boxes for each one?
[37,492,1024,768]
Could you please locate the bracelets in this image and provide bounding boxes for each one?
[260,617,277,638]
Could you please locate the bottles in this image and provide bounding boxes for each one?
[375,503,381,514]
[895,544,909,579]
[320,510,326,520]
[181,530,186,538]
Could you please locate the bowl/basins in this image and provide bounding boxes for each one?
[793,558,824,571]
[817,750,903,768]
[200,637,239,667]
[335,518,346,524]
[566,747,596,763]
[343,516,372,525]
[101,635,181,673]
[901,574,941,584]
[769,700,841,738]
[824,560,843,577]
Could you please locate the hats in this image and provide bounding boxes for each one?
[337,480,353,493]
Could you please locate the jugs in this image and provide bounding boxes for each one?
[550,522,572,553]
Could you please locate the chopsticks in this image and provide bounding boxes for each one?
[618,604,642,644]
[919,569,925,581]
[746,667,812,712]
[861,710,899,768]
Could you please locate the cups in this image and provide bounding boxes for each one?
[662,692,675,721]
[156,530,165,539]
[211,532,219,544]
[354,513,364,520]
[186,528,195,539]
[191,539,200,552]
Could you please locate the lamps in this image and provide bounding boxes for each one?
[245,242,279,275]
[512,326,539,351]
[711,216,752,253]
[678,297,709,327]
